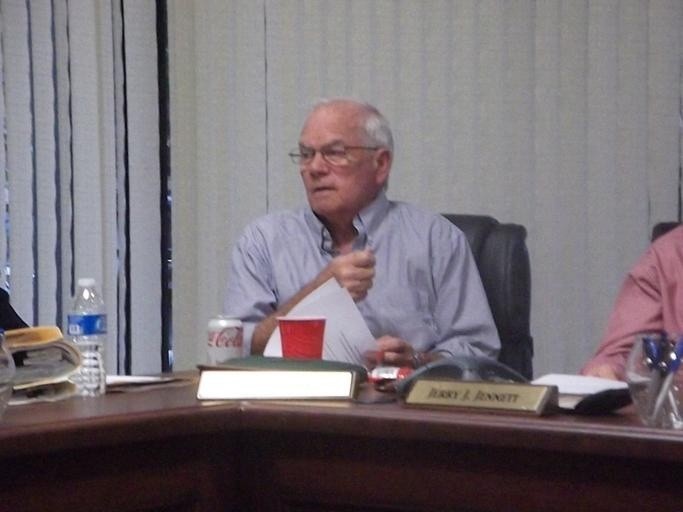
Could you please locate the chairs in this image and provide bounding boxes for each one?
[437,213,534,384]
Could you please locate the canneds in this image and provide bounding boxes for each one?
[207,314,243,364]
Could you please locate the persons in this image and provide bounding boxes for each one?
[580,223,683,412]
[231,99,503,379]
[0,289,30,365]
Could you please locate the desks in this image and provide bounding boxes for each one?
[0,368,683,511]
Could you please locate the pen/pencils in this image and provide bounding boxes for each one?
[642,331,683,426]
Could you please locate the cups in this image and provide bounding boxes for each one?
[279,316,325,361]
[625,331,683,432]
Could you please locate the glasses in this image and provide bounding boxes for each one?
[288,142,375,169]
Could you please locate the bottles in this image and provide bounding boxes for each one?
[66,278,109,399]
